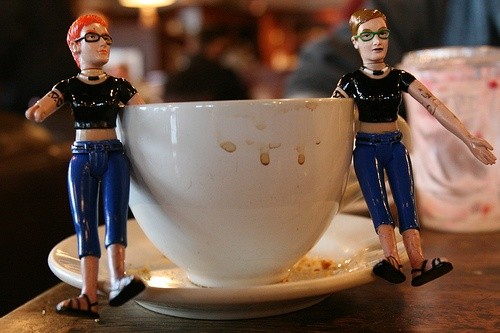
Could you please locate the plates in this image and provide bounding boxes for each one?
[47,212,410,320]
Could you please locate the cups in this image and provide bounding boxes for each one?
[113,98,356,288]
[400,46,500,235]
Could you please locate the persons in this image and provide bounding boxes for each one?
[331,8,497,287]
[25,15,144,319]
[162,23,256,103]
[291,20,360,95]
